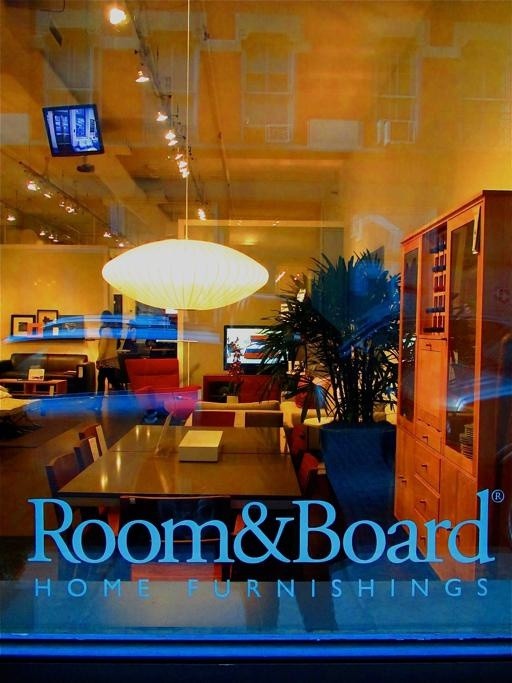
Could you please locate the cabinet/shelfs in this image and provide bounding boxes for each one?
[204,374,281,405]
[392,188,511,584]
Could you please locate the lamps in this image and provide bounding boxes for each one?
[100,0,271,316]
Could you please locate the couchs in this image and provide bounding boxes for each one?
[0,352,96,394]
[279,374,340,432]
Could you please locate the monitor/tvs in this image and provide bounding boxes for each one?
[224,324,289,375]
[42,104,105,157]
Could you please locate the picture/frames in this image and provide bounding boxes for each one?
[10,309,85,340]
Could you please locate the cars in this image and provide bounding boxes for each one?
[402,339,512,464]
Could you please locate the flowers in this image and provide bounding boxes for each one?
[224,335,245,374]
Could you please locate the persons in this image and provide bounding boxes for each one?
[122,319,138,353]
[93,310,122,416]
[145,339,156,345]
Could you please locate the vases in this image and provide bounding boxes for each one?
[228,368,242,393]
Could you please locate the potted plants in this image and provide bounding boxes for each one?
[259,248,413,528]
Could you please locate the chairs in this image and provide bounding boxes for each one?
[126,358,202,424]
[44,410,318,580]
